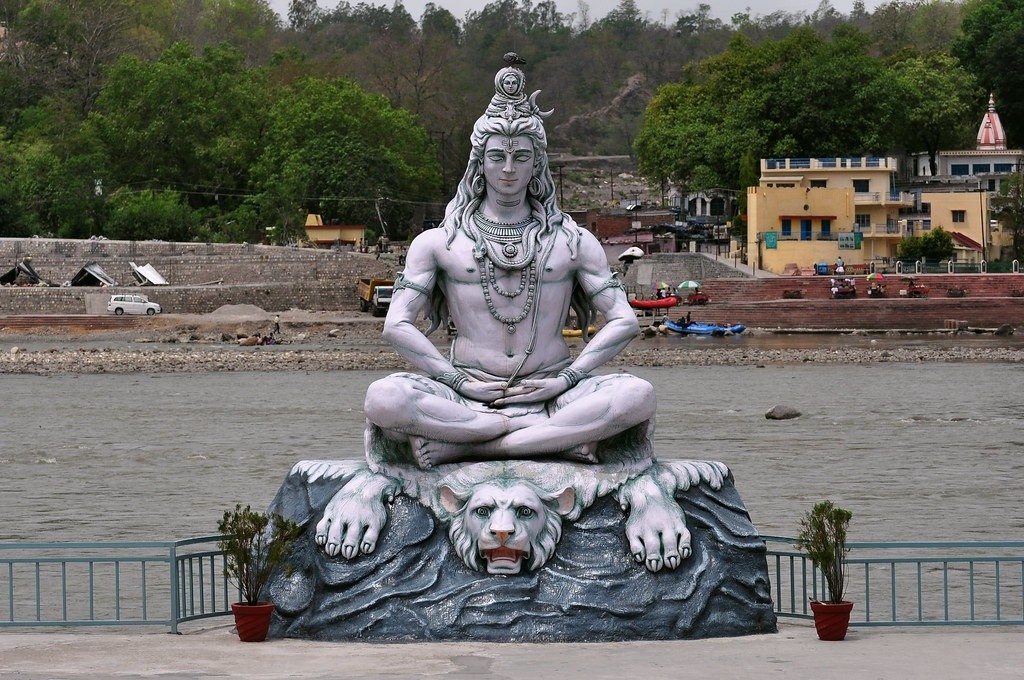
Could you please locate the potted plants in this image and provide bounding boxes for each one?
[792,499,854,641]
[217,501,304,642]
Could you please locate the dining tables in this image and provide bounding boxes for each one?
[654,284,1024,306]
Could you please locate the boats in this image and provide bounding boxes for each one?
[665,317,745,337]
[556,325,595,338]
[626,295,679,310]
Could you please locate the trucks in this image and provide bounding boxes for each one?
[356,276,397,316]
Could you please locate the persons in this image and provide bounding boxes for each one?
[836,256,845,275]
[663,285,700,297]
[662,311,697,328]
[254,313,283,346]
[357,53,663,473]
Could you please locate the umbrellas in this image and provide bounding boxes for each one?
[676,280,701,288]
[899,274,923,283]
[866,273,887,281]
[650,279,670,289]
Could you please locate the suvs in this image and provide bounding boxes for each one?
[107,294,162,316]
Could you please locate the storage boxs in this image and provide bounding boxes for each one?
[817,263,828,275]
